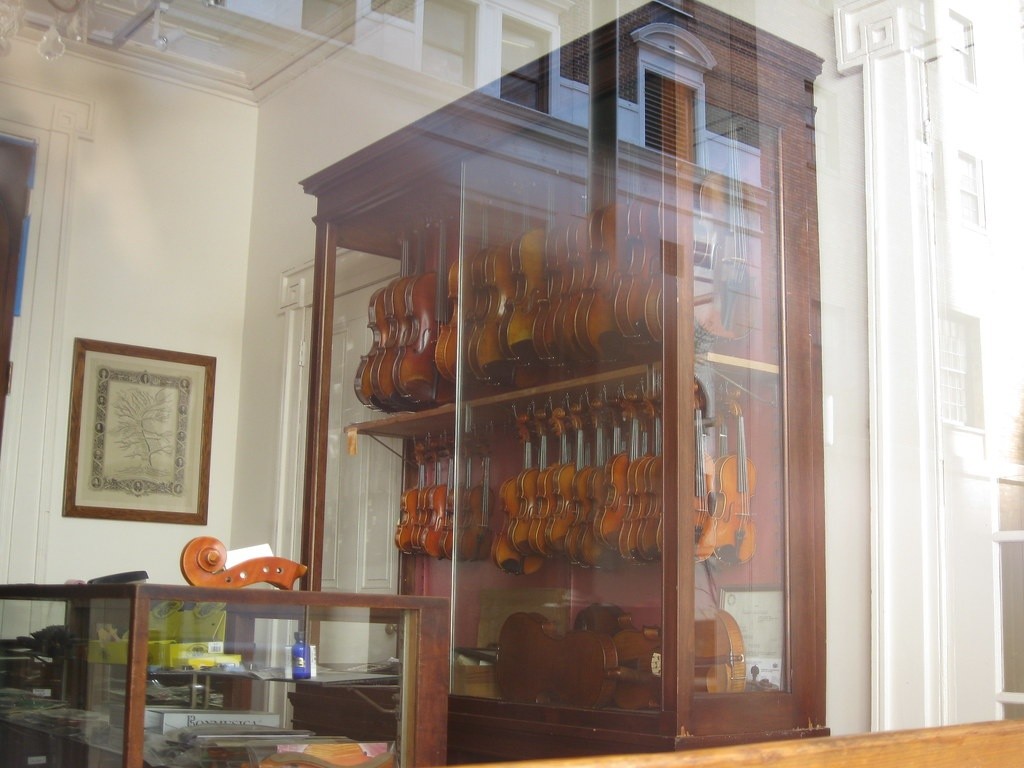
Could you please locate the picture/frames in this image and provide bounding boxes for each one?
[62,336,219,524]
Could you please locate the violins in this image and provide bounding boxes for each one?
[349,184,789,720]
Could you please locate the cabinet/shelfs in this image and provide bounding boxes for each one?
[1,583,452,768]
[288,0,830,765]
